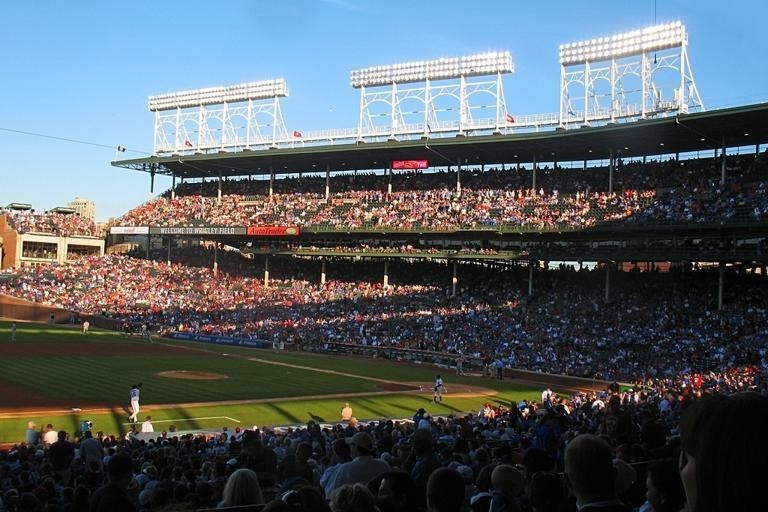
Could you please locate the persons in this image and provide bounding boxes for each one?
[2,159,768,382]
[0,353,768,512]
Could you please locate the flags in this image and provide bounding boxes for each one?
[294,131,302,137]
[568,107,575,116]
[185,140,192,147]
[506,114,514,123]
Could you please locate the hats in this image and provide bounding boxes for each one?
[346,432,375,450]
[456,465,474,479]
